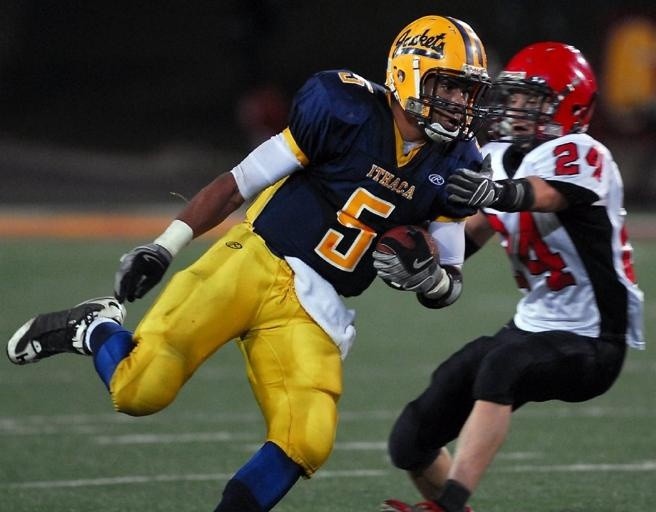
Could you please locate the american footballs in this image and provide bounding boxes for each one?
[375,226,440,265]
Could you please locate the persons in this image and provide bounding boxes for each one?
[7,14,490,511]
[379,40,646,510]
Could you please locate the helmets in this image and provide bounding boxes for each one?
[484,41,600,155]
[384,13,492,148]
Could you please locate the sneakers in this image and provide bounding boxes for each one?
[5,295,128,366]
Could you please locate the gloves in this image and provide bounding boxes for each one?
[371,228,444,296]
[445,153,506,211]
[112,242,174,304]
